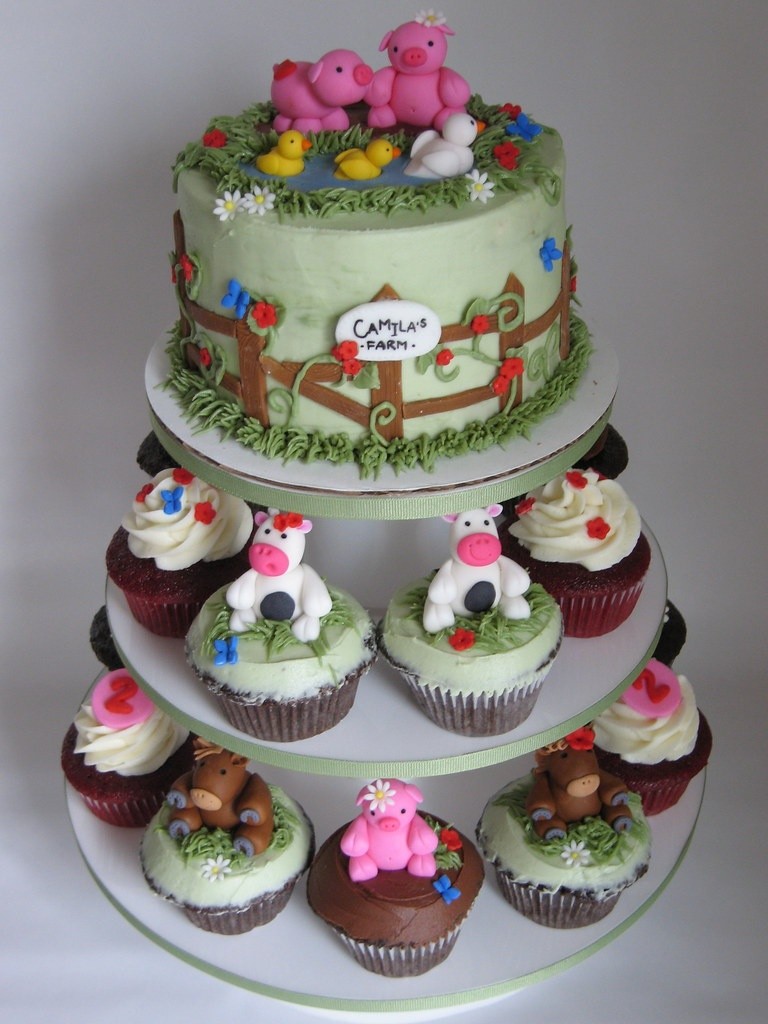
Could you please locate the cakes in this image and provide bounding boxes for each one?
[160,7,596,485]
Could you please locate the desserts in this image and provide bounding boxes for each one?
[58,424,714,978]
